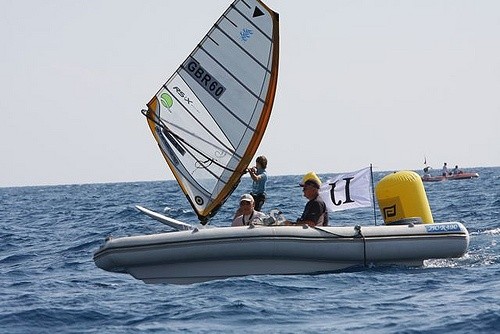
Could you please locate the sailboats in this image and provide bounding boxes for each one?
[134,0,279,231]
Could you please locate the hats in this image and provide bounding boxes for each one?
[299,179,320,189]
[238,194,254,204]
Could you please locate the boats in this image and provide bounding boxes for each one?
[93,170,469,285]
[422,173,479,182]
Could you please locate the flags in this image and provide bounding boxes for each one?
[323,166,372,212]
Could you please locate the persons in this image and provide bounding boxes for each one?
[232,155,268,225]
[452,165,463,174]
[442,162,450,176]
[231,193,266,226]
[296,179,328,227]
